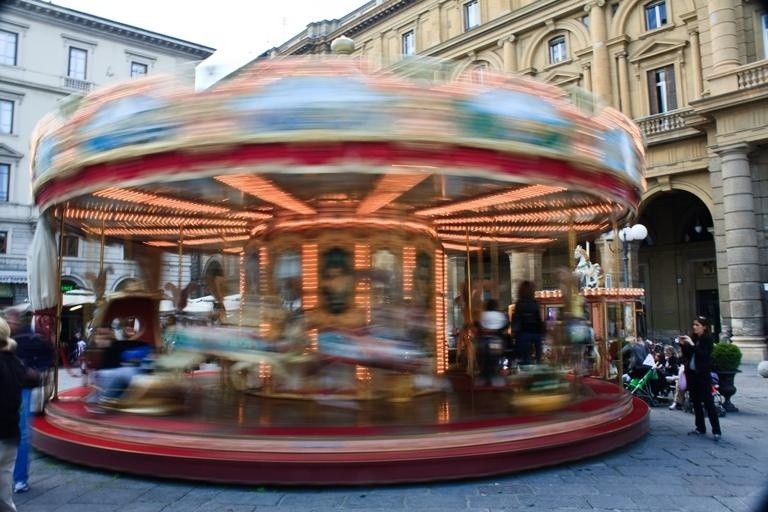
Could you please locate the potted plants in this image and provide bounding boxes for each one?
[705,343,743,411]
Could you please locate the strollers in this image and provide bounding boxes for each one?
[683,372,727,416]
[621,344,661,407]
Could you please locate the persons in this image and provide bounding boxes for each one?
[60,311,176,366]
[675,315,722,441]
[2,309,56,494]
[0,316,51,511]
[471,297,511,388]
[616,332,727,417]
[509,280,547,365]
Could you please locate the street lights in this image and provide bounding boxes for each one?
[604,224,648,288]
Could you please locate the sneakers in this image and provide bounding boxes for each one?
[668,402,675,411]
[12,482,28,493]
[688,431,703,436]
[712,433,722,440]
